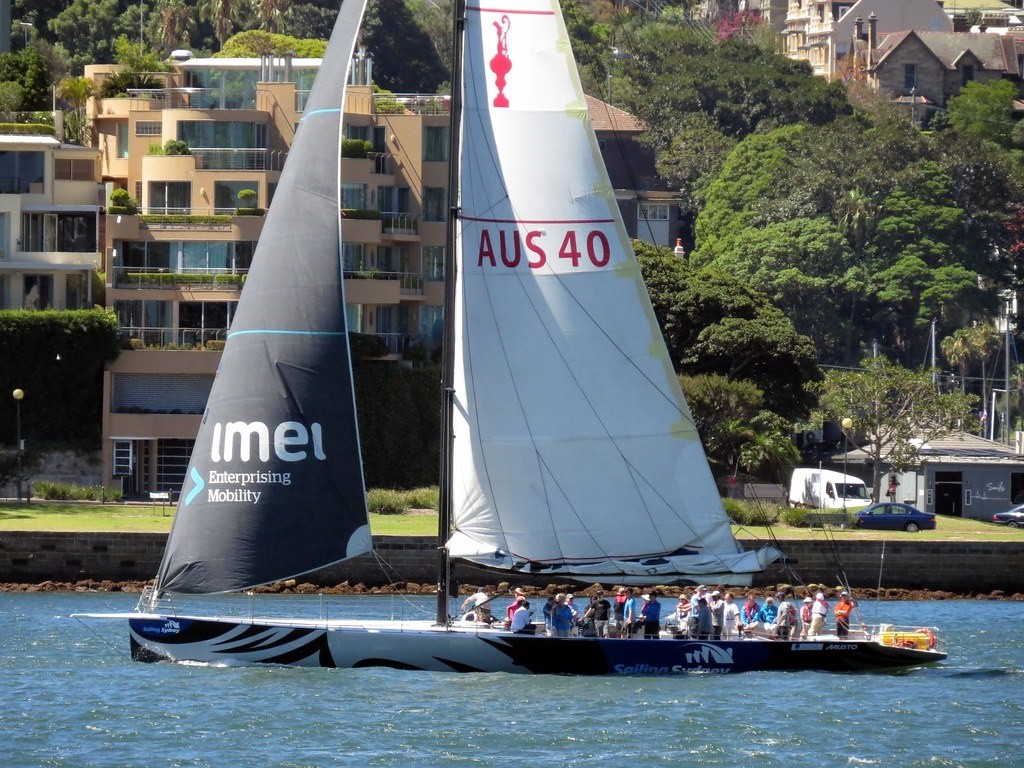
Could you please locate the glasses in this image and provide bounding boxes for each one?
[767,602,773,604]
[842,594,846,596]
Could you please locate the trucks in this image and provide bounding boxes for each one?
[789,466,873,520]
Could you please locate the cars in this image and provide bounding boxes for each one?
[851,502,937,533]
[993,502,1024,530]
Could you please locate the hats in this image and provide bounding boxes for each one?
[803,597,813,603]
[840,592,848,596]
[566,594,574,598]
[617,587,627,595]
[516,587,526,595]
[775,592,785,597]
[595,589,604,595]
[710,591,721,596]
[478,587,486,592]
[641,594,650,601]
[815,592,824,600]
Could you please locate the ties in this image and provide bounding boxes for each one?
[679,594,687,599]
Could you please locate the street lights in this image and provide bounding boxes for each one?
[13,389,25,500]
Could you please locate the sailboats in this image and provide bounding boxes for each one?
[67,0,951,673]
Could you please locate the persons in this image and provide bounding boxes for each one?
[640,591,661,639]
[510,601,537,635]
[889,474,900,503]
[550,593,575,637]
[741,596,760,637]
[639,594,650,619]
[693,598,712,640]
[708,591,724,640]
[676,593,691,639]
[565,594,579,637]
[701,586,712,611]
[543,597,556,637]
[761,596,777,638]
[508,587,526,622]
[578,590,611,638]
[807,593,828,636]
[622,587,636,638]
[799,597,814,638]
[689,585,706,639]
[680,587,696,613]
[720,592,742,640]
[785,601,798,637]
[614,588,628,638]
[773,591,790,640]
[834,591,851,638]
[461,587,493,623]
